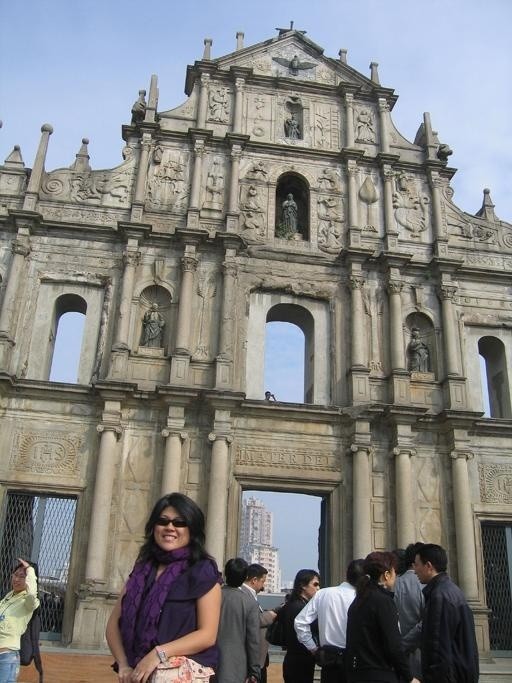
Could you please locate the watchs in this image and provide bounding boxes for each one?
[153,644,168,663]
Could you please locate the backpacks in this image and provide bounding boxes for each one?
[20,601,47,682]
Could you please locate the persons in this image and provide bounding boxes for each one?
[281,192,298,233]
[294,557,366,681]
[407,327,430,372]
[40,591,64,633]
[392,541,427,683]
[238,564,277,683]
[0,557,40,683]
[285,112,302,139]
[393,548,407,573]
[265,391,277,401]
[282,568,321,681]
[217,558,263,683]
[391,548,408,576]
[142,303,165,347]
[343,551,422,683]
[105,492,223,683]
[413,543,479,683]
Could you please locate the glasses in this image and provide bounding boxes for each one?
[307,582,323,588]
[149,516,191,528]
